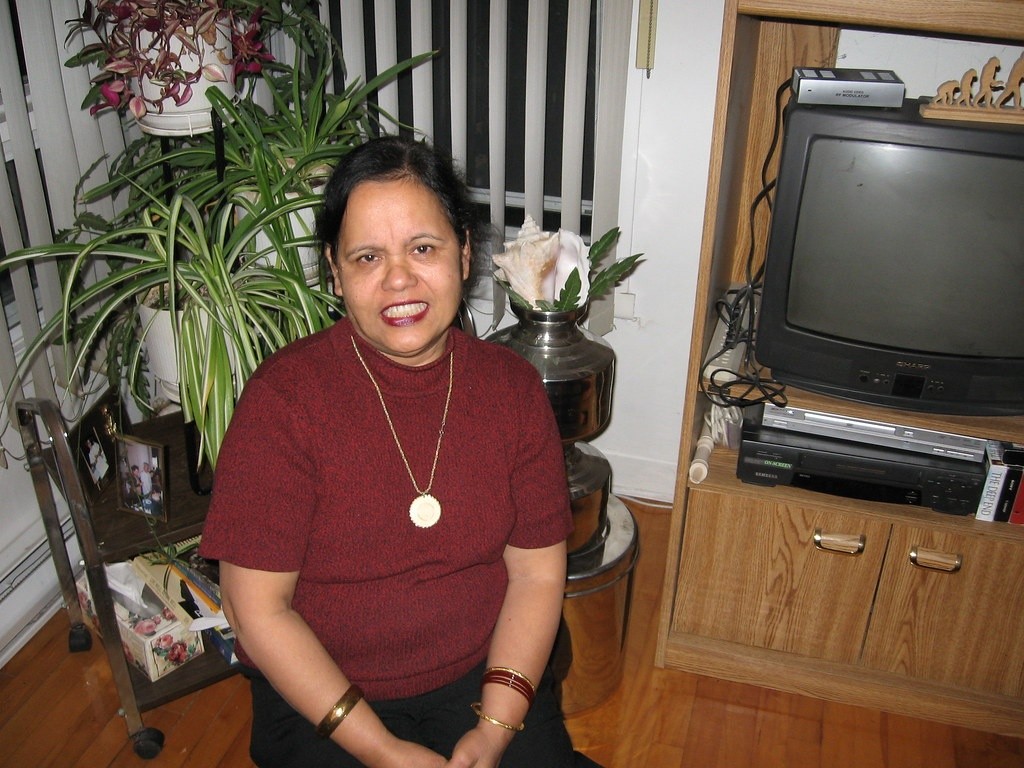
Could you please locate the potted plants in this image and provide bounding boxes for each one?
[0,0,440,473]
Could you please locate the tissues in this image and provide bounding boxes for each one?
[73,560,206,683]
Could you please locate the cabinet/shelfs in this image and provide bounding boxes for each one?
[9,391,251,758]
[653,0,1024,740]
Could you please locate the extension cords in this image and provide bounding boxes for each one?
[702,282,755,383]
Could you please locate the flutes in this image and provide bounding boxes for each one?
[687,421,715,486]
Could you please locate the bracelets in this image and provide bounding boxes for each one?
[481,667,537,706]
[313,683,364,740]
[471,701,525,731]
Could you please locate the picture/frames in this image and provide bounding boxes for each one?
[111,432,169,524]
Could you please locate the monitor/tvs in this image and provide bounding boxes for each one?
[753,97,1024,419]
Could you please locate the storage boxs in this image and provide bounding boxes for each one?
[790,66,904,108]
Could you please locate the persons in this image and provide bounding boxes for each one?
[86,439,110,480]
[126,462,152,502]
[197,135,605,768]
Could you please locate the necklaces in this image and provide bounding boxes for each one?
[350,334,454,529]
